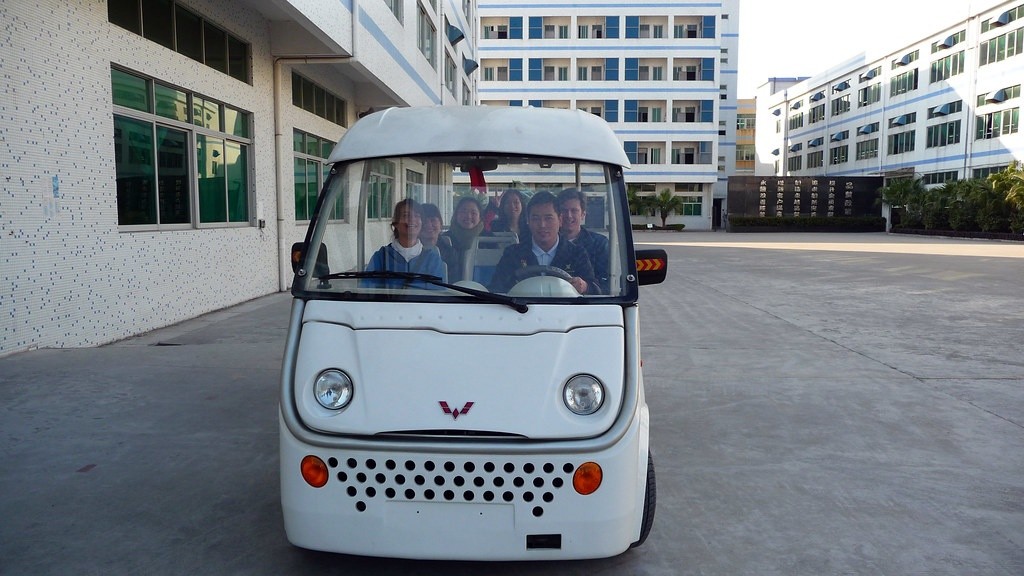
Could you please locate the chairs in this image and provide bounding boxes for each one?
[491,232,519,248]
[441,262,448,285]
[461,236,519,289]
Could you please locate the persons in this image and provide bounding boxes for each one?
[492,191,602,296]
[360,189,529,290]
[556,188,612,295]
